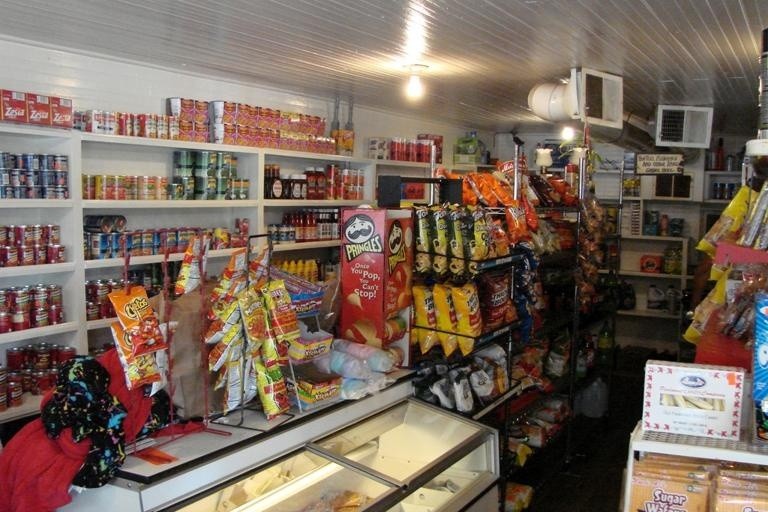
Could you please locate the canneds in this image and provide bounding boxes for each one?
[82,149,250,200]
[281,166,328,200]
[0,151,76,414]
[74,108,180,140]
[84,215,252,321]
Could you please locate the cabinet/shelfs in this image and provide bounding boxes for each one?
[529,168,742,320]
[409,204,621,482]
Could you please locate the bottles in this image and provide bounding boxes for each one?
[595,318,612,364]
[669,294,676,313]
[660,214,668,236]
[390,136,434,162]
[708,136,747,171]
[265,208,339,241]
[265,163,364,202]
